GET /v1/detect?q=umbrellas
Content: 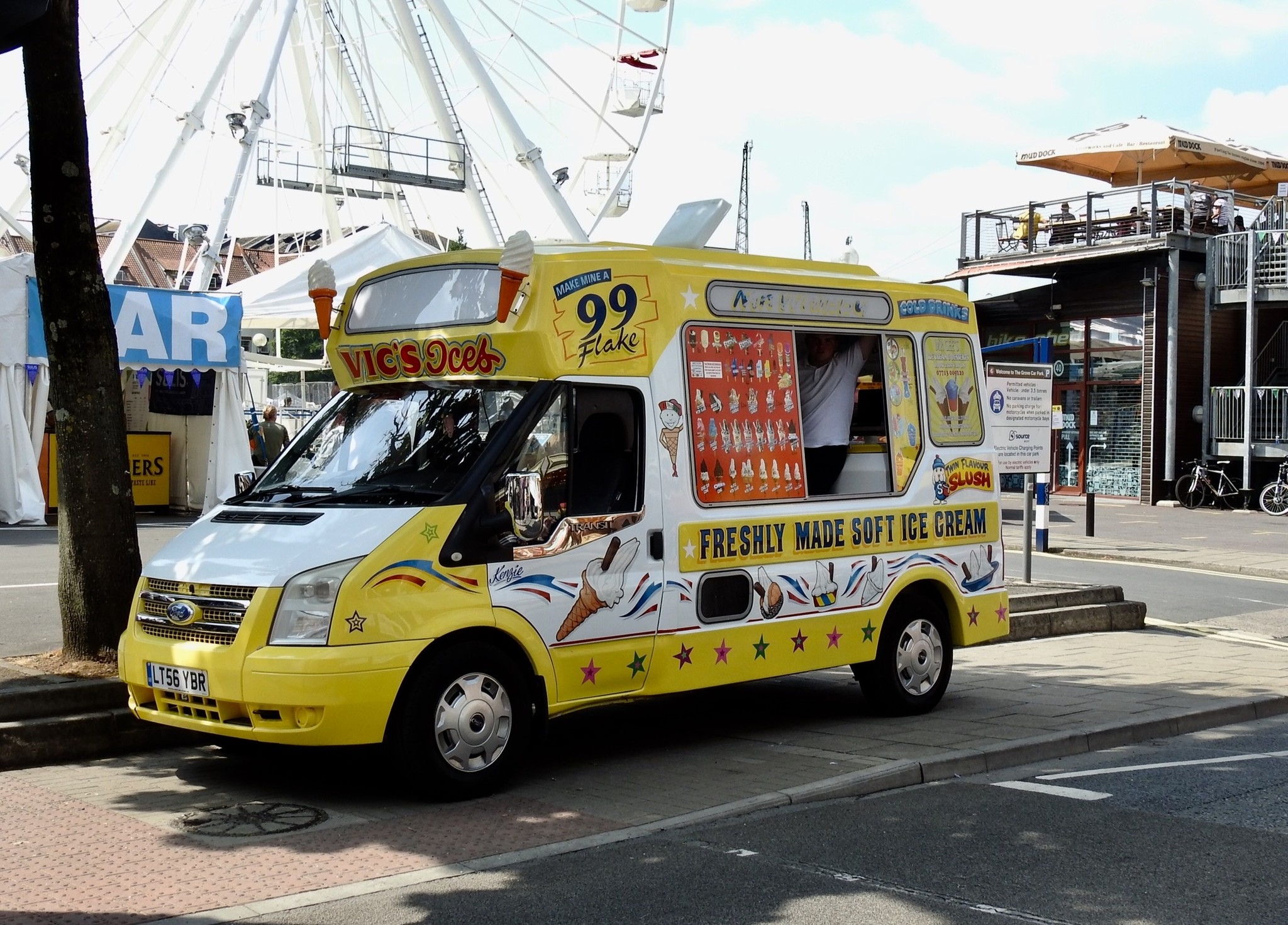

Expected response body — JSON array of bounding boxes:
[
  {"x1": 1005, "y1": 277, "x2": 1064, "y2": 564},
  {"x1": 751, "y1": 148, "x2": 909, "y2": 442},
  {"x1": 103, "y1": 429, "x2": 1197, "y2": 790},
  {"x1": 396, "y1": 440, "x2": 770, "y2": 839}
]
[{"x1": 1016, "y1": 113, "x2": 1288, "y2": 235}]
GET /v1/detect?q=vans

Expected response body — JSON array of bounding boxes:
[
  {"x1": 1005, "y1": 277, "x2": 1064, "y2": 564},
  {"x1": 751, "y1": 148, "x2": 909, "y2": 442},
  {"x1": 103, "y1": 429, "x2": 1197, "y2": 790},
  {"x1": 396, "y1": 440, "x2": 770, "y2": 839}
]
[{"x1": 118, "y1": 198, "x2": 1012, "y2": 804}]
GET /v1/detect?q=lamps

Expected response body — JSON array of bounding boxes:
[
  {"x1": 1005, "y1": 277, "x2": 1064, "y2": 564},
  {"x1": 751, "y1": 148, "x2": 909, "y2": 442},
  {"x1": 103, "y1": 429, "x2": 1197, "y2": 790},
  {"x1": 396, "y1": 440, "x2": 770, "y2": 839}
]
[
  {"x1": 1139, "y1": 278, "x2": 1155, "y2": 286},
  {"x1": 14, "y1": 153, "x2": 30, "y2": 176},
  {"x1": 552, "y1": 167, "x2": 569, "y2": 190},
  {"x1": 335, "y1": 197, "x2": 344, "y2": 210},
  {"x1": 182, "y1": 225, "x2": 209, "y2": 255},
  {"x1": 1044, "y1": 312, "x2": 1056, "y2": 321},
  {"x1": 226, "y1": 112, "x2": 248, "y2": 138},
  {"x1": 1194, "y1": 273, "x2": 1206, "y2": 290},
  {"x1": 1193, "y1": 405, "x2": 1203, "y2": 423}
]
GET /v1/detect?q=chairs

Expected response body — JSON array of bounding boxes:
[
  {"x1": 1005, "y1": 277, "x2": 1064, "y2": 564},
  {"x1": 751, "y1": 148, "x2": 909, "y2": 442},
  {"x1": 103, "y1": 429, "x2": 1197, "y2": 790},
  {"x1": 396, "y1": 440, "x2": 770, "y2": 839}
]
[
  {"x1": 996, "y1": 221, "x2": 1015, "y2": 251},
  {"x1": 484, "y1": 420, "x2": 525, "y2": 493},
  {"x1": 565, "y1": 410, "x2": 631, "y2": 515},
  {"x1": 1140, "y1": 200, "x2": 1239, "y2": 234},
  {"x1": 1049, "y1": 209, "x2": 1112, "y2": 243},
  {"x1": 1013, "y1": 220, "x2": 1037, "y2": 251}
]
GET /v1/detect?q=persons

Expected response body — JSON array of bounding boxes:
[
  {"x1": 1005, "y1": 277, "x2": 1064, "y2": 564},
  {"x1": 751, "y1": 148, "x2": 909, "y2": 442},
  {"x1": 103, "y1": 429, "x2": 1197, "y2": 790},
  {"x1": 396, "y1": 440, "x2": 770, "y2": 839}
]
[
  {"x1": 1118, "y1": 180, "x2": 1247, "y2": 237},
  {"x1": 243, "y1": 396, "x2": 292, "y2": 468},
  {"x1": 1048, "y1": 202, "x2": 1077, "y2": 246},
  {"x1": 1010, "y1": 205, "x2": 1048, "y2": 250},
  {"x1": 792, "y1": 332, "x2": 882, "y2": 498}
]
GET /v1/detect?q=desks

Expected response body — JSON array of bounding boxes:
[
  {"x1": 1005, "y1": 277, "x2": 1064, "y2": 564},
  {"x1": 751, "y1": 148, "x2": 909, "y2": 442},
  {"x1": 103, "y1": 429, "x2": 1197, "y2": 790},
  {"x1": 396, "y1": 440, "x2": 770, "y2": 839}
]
[
  {"x1": 1147, "y1": 207, "x2": 1215, "y2": 230},
  {"x1": 1038, "y1": 213, "x2": 1146, "y2": 246}
]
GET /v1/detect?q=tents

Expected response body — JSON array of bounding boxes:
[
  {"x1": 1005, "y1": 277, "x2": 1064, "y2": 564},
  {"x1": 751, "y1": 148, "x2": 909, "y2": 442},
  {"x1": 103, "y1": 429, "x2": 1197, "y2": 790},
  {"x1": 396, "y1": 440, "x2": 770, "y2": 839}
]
[{"x1": 213, "y1": 219, "x2": 441, "y2": 358}]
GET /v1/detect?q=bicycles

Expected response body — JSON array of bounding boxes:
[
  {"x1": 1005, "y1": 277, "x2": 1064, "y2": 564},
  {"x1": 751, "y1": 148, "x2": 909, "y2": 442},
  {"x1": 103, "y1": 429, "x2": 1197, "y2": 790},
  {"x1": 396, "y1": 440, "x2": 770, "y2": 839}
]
[
  {"x1": 1175, "y1": 459, "x2": 1251, "y2": 510},
  {"x1": 1259, "y1": 455, "x2": 1288, "y2": 516}
]
